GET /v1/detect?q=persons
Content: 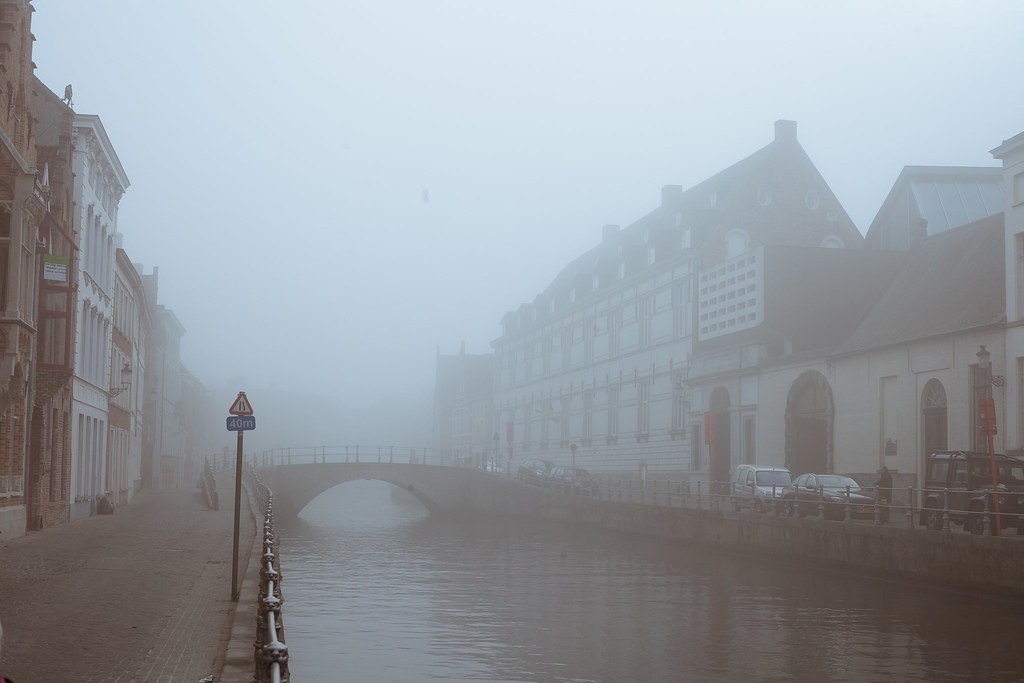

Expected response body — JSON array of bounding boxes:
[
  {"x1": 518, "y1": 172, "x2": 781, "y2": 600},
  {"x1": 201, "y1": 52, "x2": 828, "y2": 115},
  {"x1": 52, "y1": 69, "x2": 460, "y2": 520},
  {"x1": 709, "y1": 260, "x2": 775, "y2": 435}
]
[{"x1": 873, "y1": 466, "x2": 893, "y2": 522}]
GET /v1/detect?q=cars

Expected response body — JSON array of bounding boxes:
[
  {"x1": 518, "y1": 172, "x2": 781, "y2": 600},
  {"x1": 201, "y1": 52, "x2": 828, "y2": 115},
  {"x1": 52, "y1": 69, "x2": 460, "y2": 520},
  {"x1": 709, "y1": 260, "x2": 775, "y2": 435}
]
[
  {"x1": 781, "y1": 473, "x2": 876, "y2": 521},
  {"x1": 518, "y1": 458, "x2": 556, "y2": 482},
  {"x1": 550, "y1": 466, "x2": 592, "y2": 487}
]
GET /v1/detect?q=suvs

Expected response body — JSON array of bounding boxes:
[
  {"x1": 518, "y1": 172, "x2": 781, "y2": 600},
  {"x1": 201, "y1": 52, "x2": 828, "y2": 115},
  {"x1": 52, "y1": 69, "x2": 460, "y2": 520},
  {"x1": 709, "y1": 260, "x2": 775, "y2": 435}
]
[{"x1": 919, "y1": 449, "x2": 1024, "y2": 537}]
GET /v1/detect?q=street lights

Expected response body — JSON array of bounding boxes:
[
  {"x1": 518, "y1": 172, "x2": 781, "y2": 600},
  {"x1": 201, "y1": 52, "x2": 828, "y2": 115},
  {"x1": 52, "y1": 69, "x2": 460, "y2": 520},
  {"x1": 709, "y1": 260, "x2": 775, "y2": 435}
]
[{"x1": 976, "y1": 343, "x2": 1003, "y2": 537}]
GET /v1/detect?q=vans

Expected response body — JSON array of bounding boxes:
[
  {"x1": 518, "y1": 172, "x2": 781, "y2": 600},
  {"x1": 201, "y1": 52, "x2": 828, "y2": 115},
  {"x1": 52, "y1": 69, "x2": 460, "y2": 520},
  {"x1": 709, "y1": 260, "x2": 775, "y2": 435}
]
[{"x1": 731, "y1": 464, "x2": 794, "y2": 511}]
